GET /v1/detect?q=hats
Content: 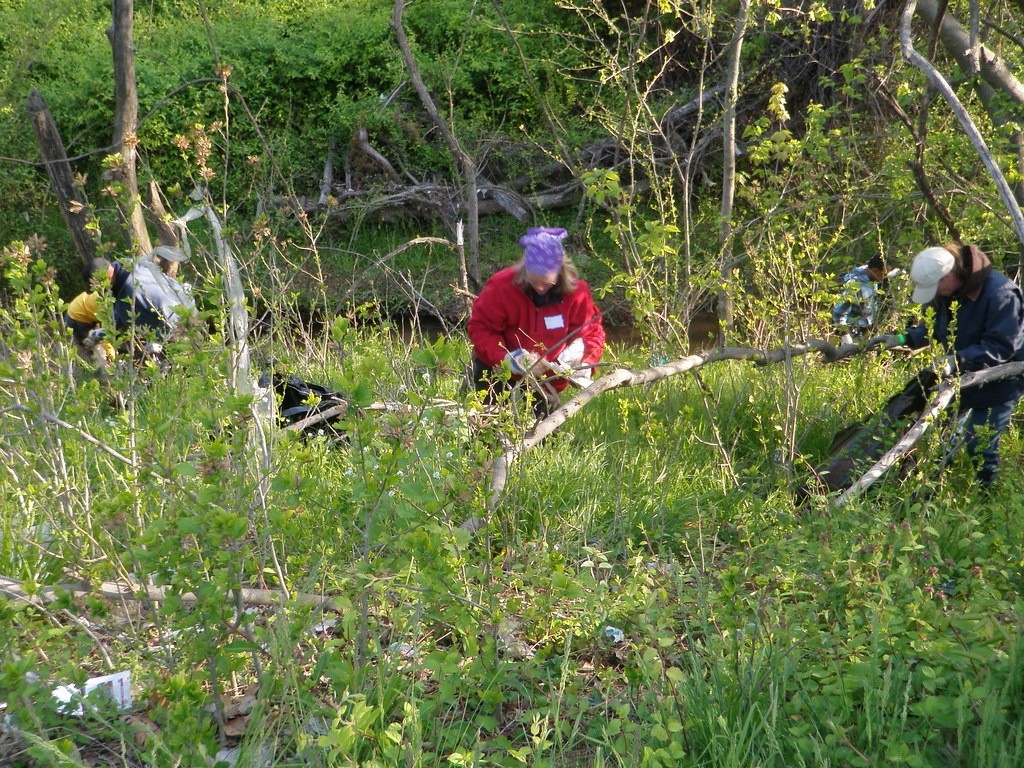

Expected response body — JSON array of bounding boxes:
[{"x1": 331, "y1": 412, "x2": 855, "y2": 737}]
[
  {"x1": 909, "y1": 248, "x2": 955, "y2": 303},
  {"x1": 84, "y1": 258, "x2": 111, "y2": 294},
  {"x1": 521, "y1": 226, "x2": 569, "y2": 272}
]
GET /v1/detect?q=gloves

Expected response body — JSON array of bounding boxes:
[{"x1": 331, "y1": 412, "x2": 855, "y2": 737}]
[
  {"x1": 867, "y1": 333, "x2": 906, "y2": 353},
  {"x1": 569, "y1": 363, "x2": 593, "y2": 390},
  {"x1": 504, "y1": 348, "x2": 547, "y2": 378},
  {"x1": 931, "y1": 355, "x2": 958, "y2": 376}
]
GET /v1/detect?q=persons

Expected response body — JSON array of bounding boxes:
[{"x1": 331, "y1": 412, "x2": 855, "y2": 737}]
[
  {"x1": 454, "y1": 226, "x2": 605, "y2": 421},
  {"x1": 64, "y1": 254, "x2": 199, "y2": 345},
  {"x1": 866, "y1": 244, "x2": 1024, "y2": 503},
  {"x1": 831, "y1": 253, "x2": 893, "y2": 339}
]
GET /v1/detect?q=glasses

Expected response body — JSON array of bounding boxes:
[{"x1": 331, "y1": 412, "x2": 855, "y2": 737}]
[{"x1": 529, "y1": 278, "x2": 557, "y2": 287}]
